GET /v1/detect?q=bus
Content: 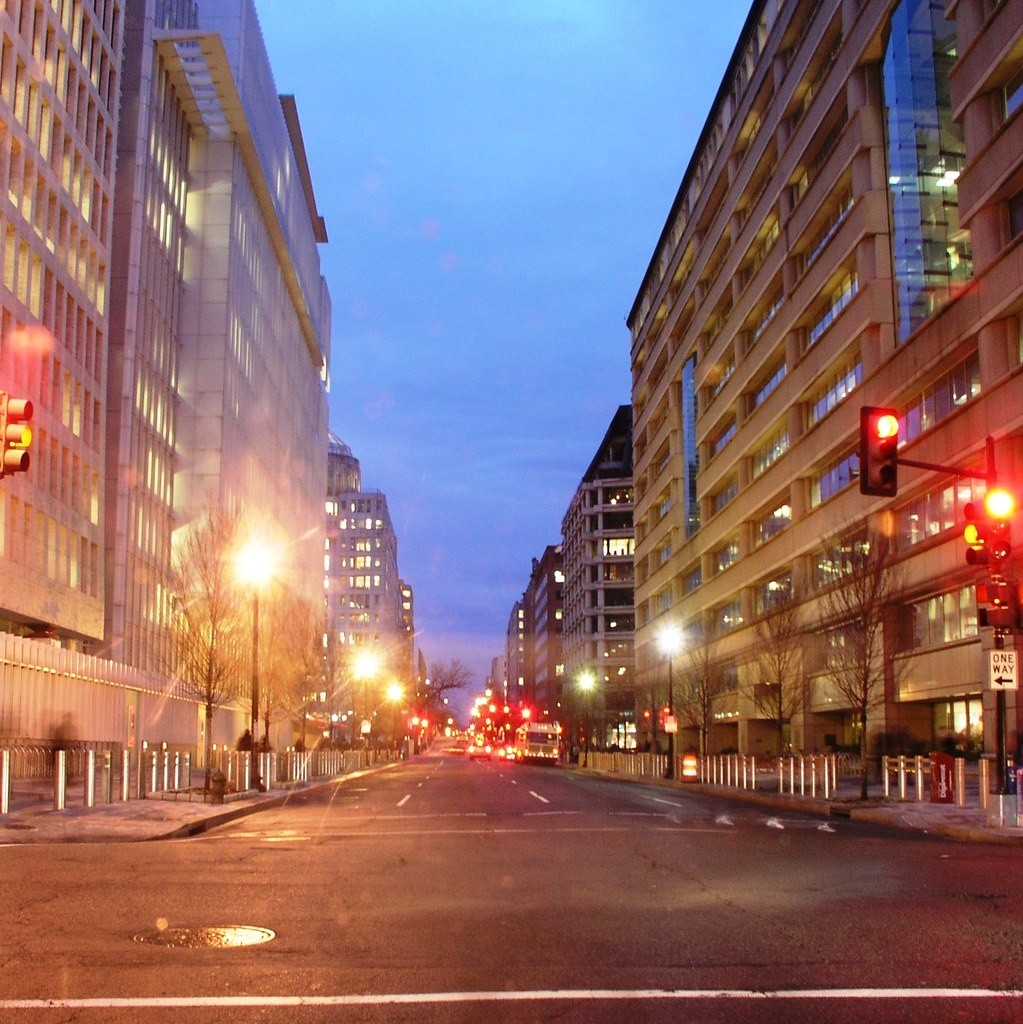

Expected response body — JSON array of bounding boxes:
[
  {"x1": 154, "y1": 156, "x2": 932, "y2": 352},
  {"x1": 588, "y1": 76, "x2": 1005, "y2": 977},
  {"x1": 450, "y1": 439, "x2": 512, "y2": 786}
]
[{"x1": 514, "y1": 722, "x2": 559, "y2": 767}]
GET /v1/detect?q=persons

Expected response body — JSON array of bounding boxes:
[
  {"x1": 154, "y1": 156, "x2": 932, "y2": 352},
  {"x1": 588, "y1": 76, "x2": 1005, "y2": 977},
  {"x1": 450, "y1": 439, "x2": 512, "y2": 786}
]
[
  {"x1": 237, "y1": 727, "x2": 426, "y2": 757},
  {"x1": 870, "y1": 725, "x2": 1023, "y2": 794},
  {"x1": 570, "y1": 738, "x2": 739, "y2": 763}
]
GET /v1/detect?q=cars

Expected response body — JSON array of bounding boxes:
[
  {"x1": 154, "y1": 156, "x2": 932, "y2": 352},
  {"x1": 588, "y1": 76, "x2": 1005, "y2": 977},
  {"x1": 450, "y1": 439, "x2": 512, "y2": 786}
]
[
  {"x1": 470, "y1": 739, "x2": 492, "y2": 761},
  {"x1": 498, "y1": 744, "x2": 516, "y2": 760}
]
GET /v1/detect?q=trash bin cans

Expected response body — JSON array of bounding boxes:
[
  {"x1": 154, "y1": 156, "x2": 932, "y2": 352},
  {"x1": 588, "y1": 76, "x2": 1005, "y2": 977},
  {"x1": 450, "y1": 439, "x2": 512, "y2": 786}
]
[{"x1": 929, "y1": 750, "x2": 955, "y2": 804}]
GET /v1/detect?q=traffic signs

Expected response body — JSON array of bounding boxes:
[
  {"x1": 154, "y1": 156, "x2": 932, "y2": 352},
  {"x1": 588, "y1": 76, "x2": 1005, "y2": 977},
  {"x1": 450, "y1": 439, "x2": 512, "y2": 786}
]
[{"x1": 988, "y1": 648, "x2": 1020, "y2": 690}]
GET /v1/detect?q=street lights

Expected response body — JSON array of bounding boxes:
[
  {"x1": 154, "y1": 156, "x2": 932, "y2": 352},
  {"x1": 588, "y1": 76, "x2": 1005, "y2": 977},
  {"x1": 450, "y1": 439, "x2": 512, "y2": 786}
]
[
  {"x1": 240, "y1": 542, "x2": 275, "y2": 790},
  {"x1": 657, "y1": 627, "x2": 681, "y2": 776},
  {"x1": 580, "y1": 672, "x2": 595, "y2": 764},
  {"x1": 357, "y1": 657, "x2": 377, "y2": 766},
  {"x1": 389, "y1": 684, "x2": 401, "y2": 742}
]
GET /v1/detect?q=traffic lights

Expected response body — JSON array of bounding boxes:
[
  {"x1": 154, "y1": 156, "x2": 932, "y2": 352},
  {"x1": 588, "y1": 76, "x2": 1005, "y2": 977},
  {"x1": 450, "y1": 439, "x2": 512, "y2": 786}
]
[
  {"x1": 984, "y1": 492, "x2": 1016, "y2": 561},
  {"x1": 0, "y1": 390, "x2": 33, "y2": 480},
  {"x1": 963, "y1": 500, "x2": 990, "y2": 569},
  {"x1": 861, "y1": 405, "x2": 901, "y2": 499},
  {"x1": 974, "y1": 579, "x2": 1022, "y2": 629}
]
